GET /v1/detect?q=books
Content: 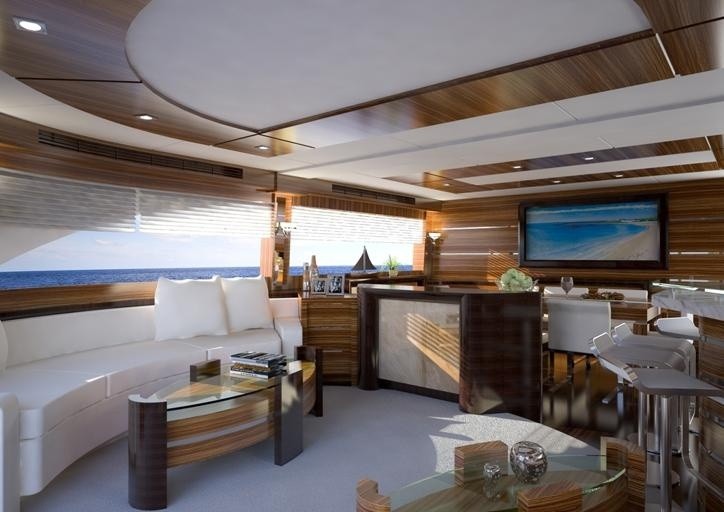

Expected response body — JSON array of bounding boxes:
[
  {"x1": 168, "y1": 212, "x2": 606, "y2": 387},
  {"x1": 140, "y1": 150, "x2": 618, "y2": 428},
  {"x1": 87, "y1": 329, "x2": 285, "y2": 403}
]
[{"x1": 228, "y1": 350, "x2": 287, "y2": 380}]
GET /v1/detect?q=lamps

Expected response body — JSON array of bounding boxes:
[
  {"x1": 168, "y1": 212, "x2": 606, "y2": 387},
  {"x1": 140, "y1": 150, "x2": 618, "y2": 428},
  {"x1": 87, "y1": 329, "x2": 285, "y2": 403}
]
[{"x1": 429, "y1": 232, "x2": 441, "y2": 275}]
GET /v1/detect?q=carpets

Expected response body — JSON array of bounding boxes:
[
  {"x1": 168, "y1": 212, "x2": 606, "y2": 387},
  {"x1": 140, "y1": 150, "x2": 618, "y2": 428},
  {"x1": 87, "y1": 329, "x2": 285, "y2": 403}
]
[{"x1": 19, "y1": 385, "x2": 682, "y2": 512}]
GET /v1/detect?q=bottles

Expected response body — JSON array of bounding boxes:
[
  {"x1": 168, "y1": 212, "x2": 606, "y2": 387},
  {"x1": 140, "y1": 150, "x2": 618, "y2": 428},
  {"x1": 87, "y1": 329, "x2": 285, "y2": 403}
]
[
  {"x1": 303, "y1": 255, "x2": 320, "y2": 291},
  {"x1": 275, "y1": 252, "x2": 285, "y2": 288}
]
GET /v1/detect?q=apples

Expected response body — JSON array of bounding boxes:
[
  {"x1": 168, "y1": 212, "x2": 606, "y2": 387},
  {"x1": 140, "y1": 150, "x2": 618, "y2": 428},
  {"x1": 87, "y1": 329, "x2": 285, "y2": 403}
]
[{"x1": 501, "y1": 268, "x2": 533, "y2": 292}]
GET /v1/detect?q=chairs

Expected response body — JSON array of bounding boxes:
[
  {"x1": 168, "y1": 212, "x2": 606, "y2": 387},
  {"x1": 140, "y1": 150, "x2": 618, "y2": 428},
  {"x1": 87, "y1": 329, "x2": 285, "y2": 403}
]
[{"x1": 547, "y1": 298, "x2": 611, "y2": 381}]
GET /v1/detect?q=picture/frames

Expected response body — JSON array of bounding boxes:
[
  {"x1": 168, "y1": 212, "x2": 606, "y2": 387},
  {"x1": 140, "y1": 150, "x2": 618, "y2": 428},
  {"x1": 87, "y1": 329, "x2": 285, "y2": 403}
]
[
  {"x1": 311, "y1": 278, "x2": 327, "y2": 294},
  {"x1": 517, "y1": 192, "x2": 670, "y2": 271},
  {"x1": 327, "y1": 273, "x2": 344, "y2": 295}
]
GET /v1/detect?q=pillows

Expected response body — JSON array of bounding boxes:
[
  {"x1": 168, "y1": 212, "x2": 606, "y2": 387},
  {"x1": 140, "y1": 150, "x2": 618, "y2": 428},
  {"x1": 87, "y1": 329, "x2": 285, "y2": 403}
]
[
  {"x1": 221, "y1": 275, "x2": 274, "y2": 333},
  {"x1": 154, "y1": 276, "x2": 228, "y2": 340}
]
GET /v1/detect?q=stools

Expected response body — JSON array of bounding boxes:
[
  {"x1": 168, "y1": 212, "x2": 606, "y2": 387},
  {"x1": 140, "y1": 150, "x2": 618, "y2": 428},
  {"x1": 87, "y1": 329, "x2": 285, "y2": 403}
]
[{"x1": 589, "y1": 316, "x2": 723, "y2": 512}]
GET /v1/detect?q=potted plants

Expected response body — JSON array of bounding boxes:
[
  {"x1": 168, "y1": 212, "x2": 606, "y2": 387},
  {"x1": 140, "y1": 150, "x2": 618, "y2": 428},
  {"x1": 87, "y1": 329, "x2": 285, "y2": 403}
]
[{"x1": 387, "y1": 256, "x2": 398, "y2": 277}]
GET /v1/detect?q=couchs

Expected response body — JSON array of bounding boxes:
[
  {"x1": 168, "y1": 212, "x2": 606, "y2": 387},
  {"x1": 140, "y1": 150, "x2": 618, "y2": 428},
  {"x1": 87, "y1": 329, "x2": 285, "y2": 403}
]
[{"x1": 0, "y1": 298, "x2": 303, "y2": 512}]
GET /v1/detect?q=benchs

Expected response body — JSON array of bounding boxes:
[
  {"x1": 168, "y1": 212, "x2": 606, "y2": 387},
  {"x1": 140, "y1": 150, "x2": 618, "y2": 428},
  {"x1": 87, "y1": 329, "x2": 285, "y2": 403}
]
[{"x1": 533, "y1": 285, "x2": 648, "y2": 302}]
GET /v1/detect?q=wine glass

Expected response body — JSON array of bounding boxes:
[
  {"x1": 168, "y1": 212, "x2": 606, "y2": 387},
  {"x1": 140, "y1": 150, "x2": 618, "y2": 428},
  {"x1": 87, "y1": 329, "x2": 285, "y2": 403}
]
[{"x1": 560, "y1": 277, "x2": 573, "y2": 298}]
